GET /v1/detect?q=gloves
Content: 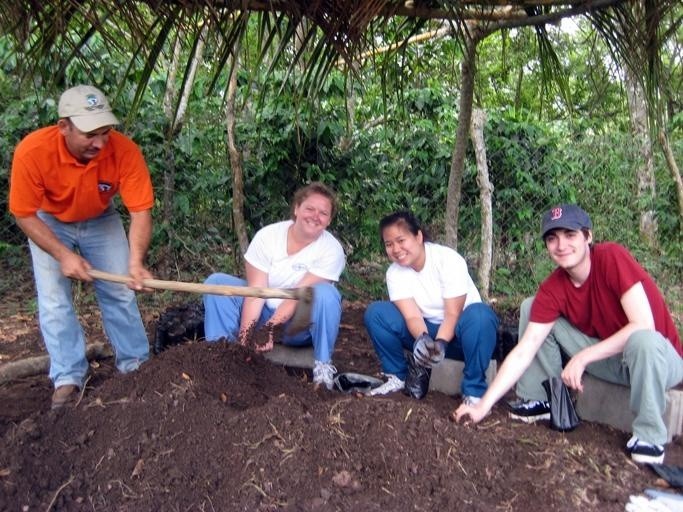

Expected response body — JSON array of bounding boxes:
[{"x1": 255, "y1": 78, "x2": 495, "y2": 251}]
[{"x1": 411, "y1": 332, "x2": 448, "y2": 370}]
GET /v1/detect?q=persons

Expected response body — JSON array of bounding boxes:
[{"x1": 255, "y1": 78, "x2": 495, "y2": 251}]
[
  {"x1": 6, "y1": 83, "x2": 164, "y2": 408},
  {"x1": 201, "y1": 181, "x2": 346, "y2": 391},
  {"x1": 361, "y1": 210, "x2": 500, "y2": 417},
  {"x1": 451, "y1": 203, "x2": 683, "y2": 466}
]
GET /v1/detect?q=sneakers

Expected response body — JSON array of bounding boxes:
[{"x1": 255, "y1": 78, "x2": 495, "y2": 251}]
[
  {"x1": 50, "y1": 385, "x2": 81, "y2": 409},
  {"x1": 626, "y1": 434, "x2": 665, "y2": 464},
  {"x1": 312, "y1": 361, "x2": 337, "y2": 401},
  {"x1": 452, "y1": 395, "x2": 492, "y2": 420},
  {"x1": 364, "y1": 371, "x2": 407, "y2": 404},
  {"x1": 507, "y1": 398, "x2": 551, "y2": 424}
]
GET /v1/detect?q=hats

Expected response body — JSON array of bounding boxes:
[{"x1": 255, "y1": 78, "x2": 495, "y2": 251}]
[
  {"x1": 540, "y1": 206, "x2": 590, "y2": 240},
  {"x1": 57, "y1": 84, "x2": 120, "y2": 134}
]
